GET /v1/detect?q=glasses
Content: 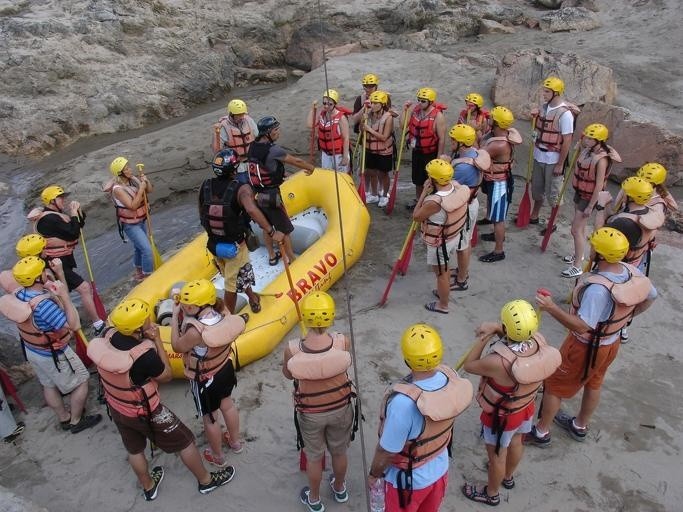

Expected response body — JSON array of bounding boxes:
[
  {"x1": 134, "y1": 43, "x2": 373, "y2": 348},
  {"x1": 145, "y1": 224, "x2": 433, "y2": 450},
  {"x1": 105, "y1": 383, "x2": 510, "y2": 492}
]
[
  {"x1": 323, "y1": 102, "x2": 333, "y2": 105},
  {"x1": 417, "y1": 98, "x2": 428, "y2": 103}
]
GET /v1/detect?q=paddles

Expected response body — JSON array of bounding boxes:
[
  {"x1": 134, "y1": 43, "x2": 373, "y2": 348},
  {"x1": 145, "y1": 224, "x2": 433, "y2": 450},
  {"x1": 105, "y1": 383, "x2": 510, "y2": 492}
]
[
  {"x1": 76, "y1": 211, "x2": 107, "y2": 322},
  {"x1": 516, "y1": 112, "x2": 537, "y2": 227},
  {"x1": 54, "y1": 294, "x2": 94, "y2": 371},
  {"x1": 387, "y1": 107, "x2": 408, "y2": 214},
  {"x1": 140, "y1": 170, "x2": 162, "y2": 272},
  {"x1": 358, "y1": 108, "x2": 368, "y2": 205},
  {"x1": 542, "y1": 136, "x2": 581, "y2": 251},
  {"x1": 400, "y1": 184, "x2": 430, "y2": 274},
  {"x1": 1, "y1": 371, "x2": 26, "y2": 413}
]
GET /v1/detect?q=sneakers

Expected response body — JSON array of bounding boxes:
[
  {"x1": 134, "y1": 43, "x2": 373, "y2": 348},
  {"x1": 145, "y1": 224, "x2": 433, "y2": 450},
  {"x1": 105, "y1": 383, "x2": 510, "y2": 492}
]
[
  {"x1": 198, "y1": 465, "x2": 235, "y2": 495},
  {"x1": 406, "y1": 198, "x2": 418, "y2": 210},
  {"x1": 4, "y1": 421, "x2": 25, "y2": 444},
  {"x1": 366, "y1": 194, "x2": 379, "y2": 204},
  {"x1": 480, "y1": 232, "x2": 504, "y2": 242},
  {"x1": 562, "y1": 253, "x2": 585, "y2": 264},
  {"x1": 540, "y1": 223, "x2": 558, "y2": 236},
  {"x1": 203, "y1": 447, "x2": 228, "y2": 468},
  {"x1": 300, "y1": 485, "x2": 326, "y2": 512},
  {"x1": 89, "y1": 324, "x2": 114, "y2": 338},
  {"x1": 69, "y1": 414, "x2": 103, "y2": 434},
  {"x1": 620, "y1": 326, "x2": 632, "y2": 344},
  {"x1": 480, "y1": 252, "x2": 506, "y2": 263},
  {"x1": 142, "y1": 464, "x2": 164, "y2": 502},
  {"x1": 560, "y1": 266, "x2": 583, "y2": 278},
  {"x1": 477, "y1": 217, "x2": 492, "y2": 225},
  {"x1": 378, "y1": 191, "x2": 391, "y2": 199},
  {"x1": 377, "y1": 196, "x2": 389, "y2": 208},
  {"x1": 248, "y1": 293, "x2": 262, "y2": 313},
  {"x1": 329, "y1": 472, "x2": 350, "y2": 503},
  {"x1": 60, "y1": 406, "x2": 86, "y2": 430},
  {"x1": 223, "y1": 430, "x2": 244, "y2": 454},
  {"x1": 364, "y1": 191, "x2": 376, "y2": 197},
  {"x1": 514, "y1": 216, "x2": 540, "y2": 225},
  {"x1": 134, "y1": 272, "x2": 144, "y2": 281},
  {"x1": 237, "y1": 312, "x2": 250, "y2": 324},
  {"x1": 553, "y1": 413, "x2": 588, "y2": 443},
  {"x1": 521, "y1": 426, "x2": 551, "y2": 449}
]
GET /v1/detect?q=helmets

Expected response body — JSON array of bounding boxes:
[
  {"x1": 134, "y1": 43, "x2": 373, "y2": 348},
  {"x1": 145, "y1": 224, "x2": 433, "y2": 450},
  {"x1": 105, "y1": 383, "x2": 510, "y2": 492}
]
[
  {"x1": 226, "y1": 98, "x2": 248, "y2": 115},
  {"x1": 324, "y1": 90, "x2": 340, "y2": 105},
  {"x1": 209, "y1": 148, "x2": 239, "y2": 176},
  {"x1": 255, "y1": 114, "x2": 281, "y2": 134},
  {"x1": 110, "y1": 155, "x2": 129, "y2": 178},
  {"x1": 402, "y1": 323, "x2": 444, "y2": 372},
  {"x1": 543, "y1": 76, "x2": 565, "y2": 96},
  {"x1": 369, "y1": 90, "x2": 389, "y2": 105},
  {"x1": 13, "y1": 256, "x2": 46, "y2": 288},
  {"x1": 621, "y1": 176, "x2": 654, "y2": 205},
  {"x1": 465, "y1": 92, "x2": 485, "y2": 108},
  {"x1": 416, "y1": 87, "x2": 437, "y2": 102},
  {"x1": 425, "y1": 158, "x2": 455, "y2": 186},
  {"x1": 111, "y1": 295, "x2": 150, "y2": 336},
  {"x1": 360, "y1": 72, "x2": 381, "y2": 87},
  {"x1": 449, "y1": 124, "x2": 476, "y2": 147},
  {"x1": 179, "y1": 279, "x2": 218, "y2": 307},
  {"x1": 583, "y1": 122, "x2": 609, "y2": 143},
  {"x1": 590, "y1": 228, "x2": 630, "y2": 264},
  {"x1": 41, "y1": 183, "x2": 71, "y2": 204},
  {"x1": 300, "y1": 290, "x2": 338, "y2": 329},
  {"x1": 501, "y1": 299, "x2": 539, "y2": 342},
  {"x1": 637, "y1": 162, "x2": 667, "y2": 185},
  {"x1": 491, "y1": 106, "x2": 513, "y2": 129},
  {"x1": 16, "y1": 232, "x2": 46, "y2": 258}
]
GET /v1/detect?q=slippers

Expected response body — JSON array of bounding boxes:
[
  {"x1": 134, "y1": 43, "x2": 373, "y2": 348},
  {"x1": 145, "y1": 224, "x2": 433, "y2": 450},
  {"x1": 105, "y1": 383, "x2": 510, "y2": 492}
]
[
  {"x1": 425, "y1": 302, "x2": 449, "y2": 314},
  {"x1": 432, "y1": 288, "x2": 440, "y2": 299}
]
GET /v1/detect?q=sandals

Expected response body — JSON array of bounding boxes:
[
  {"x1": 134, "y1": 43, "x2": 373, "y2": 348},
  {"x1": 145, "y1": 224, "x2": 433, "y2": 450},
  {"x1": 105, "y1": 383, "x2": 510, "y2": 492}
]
[
  {"x1": 448, "y1": 277, "x2": 468, "y2": 291},
  {"x1": 462, "y1": 483, "x2": 502, "y2": 507},
  {"x1": 269, "y1": 251, "x2": 282, "y2": 266},
  {"x1": 448, "y1": 266, "x2": 470, "y2": 279},
  {"x1": 486, "y1": 460, "x2": 515, "y2": 490}
]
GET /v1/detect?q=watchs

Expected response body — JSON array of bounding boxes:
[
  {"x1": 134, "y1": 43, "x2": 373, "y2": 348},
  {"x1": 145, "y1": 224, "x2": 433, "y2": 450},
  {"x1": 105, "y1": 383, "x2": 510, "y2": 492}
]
[
  {"x1": 268, "y1": 225, "x2": 276, "y2": 238},
  {"x1": 596, "y1": 203, "x2": 605, "y2": 211}
]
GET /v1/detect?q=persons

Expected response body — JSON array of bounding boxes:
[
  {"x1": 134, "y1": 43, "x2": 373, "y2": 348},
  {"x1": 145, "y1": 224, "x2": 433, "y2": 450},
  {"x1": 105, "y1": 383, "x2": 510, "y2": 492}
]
[
  {"x1": 102, "y1": 156, "x2": 154, "y2": 282},
  {"x1": 213, "y1": 99, "x2": 259, "y2": 184},
  {"x1": 359, "y1": 90, "x2": 400, "y2": 207},
  {"x1": 351, "y1": 73, "x2": 392, "y2": 199},
  {"x1": 412, "y1": 158, "x2": 470, "y2": 314},
  {"x1": 457, "y1": 93, "x2": 483, "y2": 127},
  {"x1": 170, "y1": 278, "x2": 245, "y2": 468},
  {"x1": 474, "y1": 106, "x2": 495, "y2": 225},
  {"x1": 462, "y1": 299, "x2": 562, "y2": 506},
  {"x1": 198, "y1": 147, "x2": 285, "y2": 323},
  {"x1": 521, "y1": 227, "x2": 658, "y2": 450},
  {"x1": 438, "y1": 126, "x2": 490, "y2": 291},
  {"x1": 594, "y1": 178, "x2": 665, "y2": 344},
  {"x1": 87, "y1": 298, "x2": 236, "y2": 502},
  {"x1": 615, "y1": 163, "x2": 677, "y2": 213},
  {"x1": 560, "y1": 123, "x2": 622, "y2": 278},
  {"x1": 512, "y1": 76, "x2": 582, "y2": 233},
  {"x1": 306, "y1": 88, "x2": 353, "y2": 175},
  {"x1": 27, "y1": 185, "x2": 106, "y2": 336},
  {"x1": 0, "y1": 232, "x2": 69, "y2": 297},
  {"x1": 283, "y1": 290, "x2": 359, "y2": 512},
  {"x1": 399, "y1": 87, "x2": 447, "y2": 209},
  {"x1": 0, "y1": 256, "x2": 103, "y2": 434},
  {"x1": 246, "y1": 115, "x2": 314, "y2": 266},
  {"x1": 478, "y1": 105, "x2": 522, "y2": 262},
  {"x1": 0, "y1": 382, "x2": 26, "y2": 444},
  {"x1": 367, "y1": 321, "x2": 473, "y2": 512}
]
